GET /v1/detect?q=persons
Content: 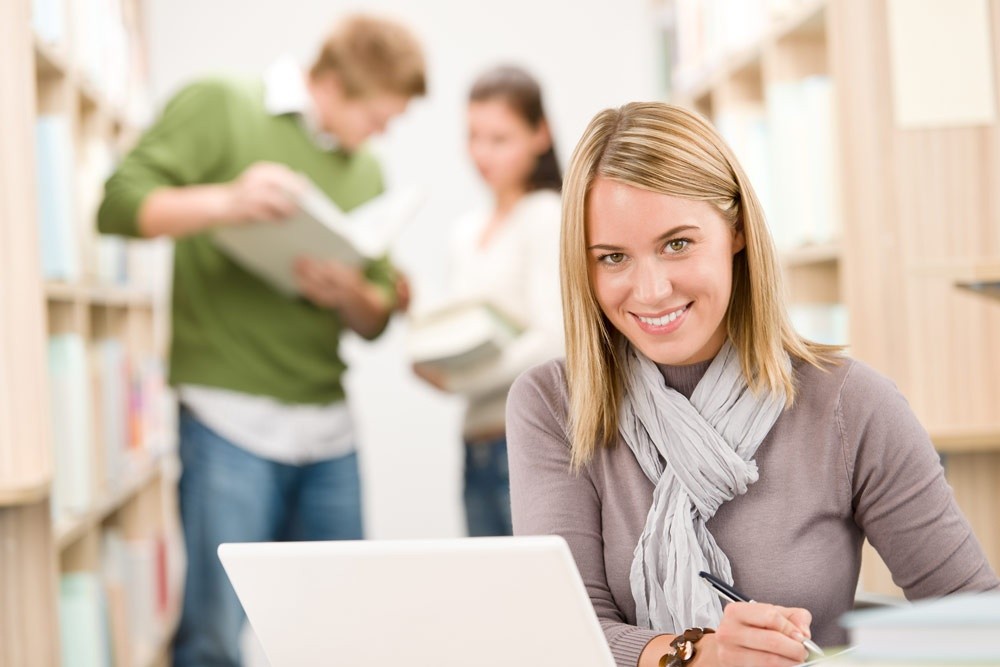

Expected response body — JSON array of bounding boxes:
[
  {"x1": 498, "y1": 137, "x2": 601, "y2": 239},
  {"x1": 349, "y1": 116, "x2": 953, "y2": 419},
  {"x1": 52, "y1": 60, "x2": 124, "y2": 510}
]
[
  {"x1": 394, "y1": 64, "x2": 570, "y2": 538},
  {"x1": 504, "y1": 102, "x2": 1000, "y2": 667},
  {"x1": 96, "y1": 17, "x2": 430, "y2": 667}
]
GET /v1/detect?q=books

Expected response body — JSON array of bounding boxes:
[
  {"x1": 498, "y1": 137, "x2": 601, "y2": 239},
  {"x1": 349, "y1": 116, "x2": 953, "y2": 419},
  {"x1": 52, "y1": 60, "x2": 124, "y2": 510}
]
[
  {"x1": 216, "y1": 159, "x2": 387, "y2": 303},
  {"x1": 405, "y1": 284, "x2": 529, "y2": 388},
  {"x1": 840, "y1": 593, "x2": 1000, "y2": 663}
]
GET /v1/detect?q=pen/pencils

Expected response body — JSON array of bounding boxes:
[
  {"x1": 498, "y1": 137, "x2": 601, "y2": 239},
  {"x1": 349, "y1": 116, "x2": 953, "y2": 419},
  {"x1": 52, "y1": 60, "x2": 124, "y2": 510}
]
[{"x1": 699, "y1": 572, "x2": 825, "y2": 656}]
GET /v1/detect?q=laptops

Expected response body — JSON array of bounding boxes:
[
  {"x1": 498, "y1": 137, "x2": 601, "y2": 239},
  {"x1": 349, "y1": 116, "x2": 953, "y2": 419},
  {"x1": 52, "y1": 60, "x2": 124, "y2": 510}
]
[{"x1": 216, "y1": 533, "x2": 618, "y2": 667}]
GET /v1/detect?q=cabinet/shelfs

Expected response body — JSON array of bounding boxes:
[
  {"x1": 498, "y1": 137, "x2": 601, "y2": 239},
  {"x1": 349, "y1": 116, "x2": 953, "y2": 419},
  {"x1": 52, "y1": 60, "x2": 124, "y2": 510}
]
[
  {"x1": 654, "y1": 0, "x2": 1000, "y2": 606},
  {"x1": 0, "y1": 1, "x2": 197, "y2": 667}
]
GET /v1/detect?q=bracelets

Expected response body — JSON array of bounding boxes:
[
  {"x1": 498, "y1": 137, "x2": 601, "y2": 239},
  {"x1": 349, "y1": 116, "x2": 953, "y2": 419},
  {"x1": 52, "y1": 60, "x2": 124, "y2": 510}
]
[{"x1": 657, "y1": 626, "x2": 715, "y2": 667}]
